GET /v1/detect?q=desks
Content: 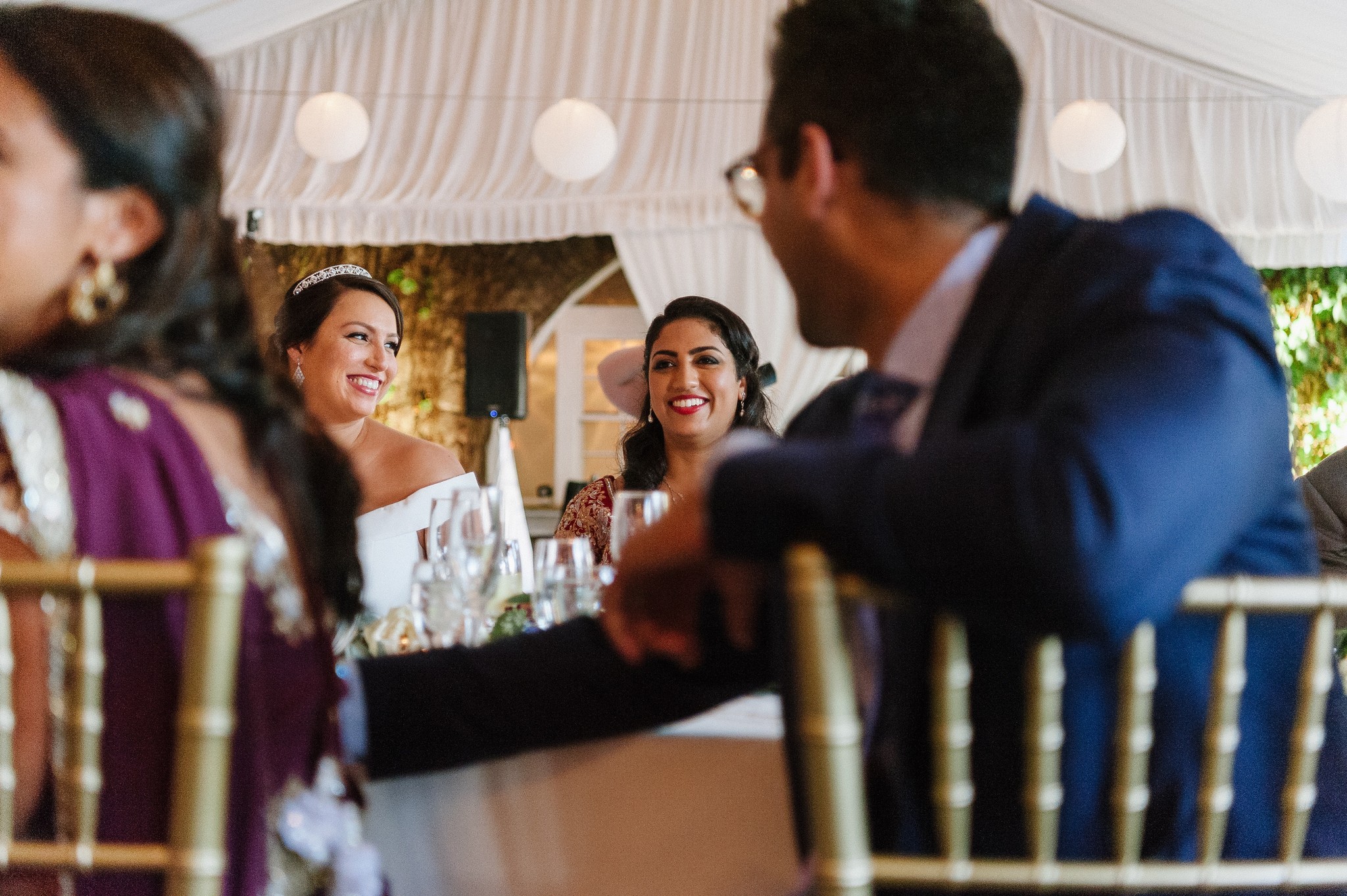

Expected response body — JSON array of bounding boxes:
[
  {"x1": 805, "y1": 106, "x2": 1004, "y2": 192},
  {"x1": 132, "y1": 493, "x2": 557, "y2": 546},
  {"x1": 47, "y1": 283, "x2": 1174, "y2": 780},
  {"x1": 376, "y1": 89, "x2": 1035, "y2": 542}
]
[{"x1": 362, "y1": 693, "x2": 802, "y2": 896}]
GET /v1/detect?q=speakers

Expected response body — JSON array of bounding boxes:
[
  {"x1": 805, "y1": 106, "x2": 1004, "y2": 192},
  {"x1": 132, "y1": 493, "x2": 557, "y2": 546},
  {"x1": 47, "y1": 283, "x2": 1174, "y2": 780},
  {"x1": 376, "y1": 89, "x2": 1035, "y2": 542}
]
[{"x1": 463, "y1": 310, "x2": 528, "y2": 420}]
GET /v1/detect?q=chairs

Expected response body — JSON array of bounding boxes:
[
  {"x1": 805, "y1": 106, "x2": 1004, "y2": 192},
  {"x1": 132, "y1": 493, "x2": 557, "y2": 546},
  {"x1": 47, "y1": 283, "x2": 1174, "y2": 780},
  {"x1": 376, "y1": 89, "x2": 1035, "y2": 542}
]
[
  {"x1": 789, "y1": 543, "x2": 1347, "y2": 896},
  {"x1": 0, "y1": 533, "x2": 245, "y2": 896}
]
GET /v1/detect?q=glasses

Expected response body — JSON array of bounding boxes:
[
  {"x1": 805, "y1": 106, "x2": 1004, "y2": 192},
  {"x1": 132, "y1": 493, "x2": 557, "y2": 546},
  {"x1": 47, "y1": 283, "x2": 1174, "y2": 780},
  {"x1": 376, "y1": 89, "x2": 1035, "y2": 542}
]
[{"x1": 723, "y1": 131, "x2": 844, "y2": 222}]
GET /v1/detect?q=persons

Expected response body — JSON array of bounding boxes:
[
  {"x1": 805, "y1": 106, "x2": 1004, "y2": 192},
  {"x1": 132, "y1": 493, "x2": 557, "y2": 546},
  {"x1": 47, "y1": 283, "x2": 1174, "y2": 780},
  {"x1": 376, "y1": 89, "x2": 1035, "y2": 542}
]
[
  {"x1": 551, "y1": 296, "x2": 783, "y2": 620},
  {"x1": 1, "y1": 0, "x2": 394, "y2": 896},
  {"x1": 267, "y1": 264, "x2": 503, "y2": 643},
  {"x1": 350, "y1": 0, "x2": 1347, "y2": 896},
  {"x1": 1290, "y1": 450, "x2": 1347, "y2": 631}
]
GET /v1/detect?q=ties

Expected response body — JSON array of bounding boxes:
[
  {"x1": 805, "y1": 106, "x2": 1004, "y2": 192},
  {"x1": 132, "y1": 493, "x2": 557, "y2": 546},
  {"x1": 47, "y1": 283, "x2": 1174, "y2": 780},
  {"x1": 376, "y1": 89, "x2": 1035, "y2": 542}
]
[{"x1": 836, "y1": 375, "x2": 914, "y2": 737}]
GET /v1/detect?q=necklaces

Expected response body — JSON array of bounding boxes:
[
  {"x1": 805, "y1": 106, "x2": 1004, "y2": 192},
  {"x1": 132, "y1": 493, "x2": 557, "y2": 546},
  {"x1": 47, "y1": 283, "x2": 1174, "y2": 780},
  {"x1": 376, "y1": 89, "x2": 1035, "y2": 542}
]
[{"x1": 660, "y1": 475, "x2": 684, "y2": 506}]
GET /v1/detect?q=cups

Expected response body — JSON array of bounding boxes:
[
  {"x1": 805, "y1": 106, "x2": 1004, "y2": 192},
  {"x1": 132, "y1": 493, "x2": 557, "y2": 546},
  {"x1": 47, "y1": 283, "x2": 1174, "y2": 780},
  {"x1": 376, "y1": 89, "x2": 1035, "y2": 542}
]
[
  {"x1": 425, "y1": 496, "x2": 450, "y2": 578},
  {"x1": 610, "y1": 490, "x2": 671, "y2": 561},
  {"x1": 533, "y1": 540, "x2": 602, "y2": 631},
  {"x1": 409, "y1": 557, "x2": 475, "y2": 649}
]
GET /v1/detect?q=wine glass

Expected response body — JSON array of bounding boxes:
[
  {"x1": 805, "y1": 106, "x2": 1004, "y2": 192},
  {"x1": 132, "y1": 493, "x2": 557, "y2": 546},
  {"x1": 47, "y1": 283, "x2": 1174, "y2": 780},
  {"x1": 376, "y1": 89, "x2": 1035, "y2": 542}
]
[{"x1": 450, "y1": 484, "x2": 504, "y2": 648}]
